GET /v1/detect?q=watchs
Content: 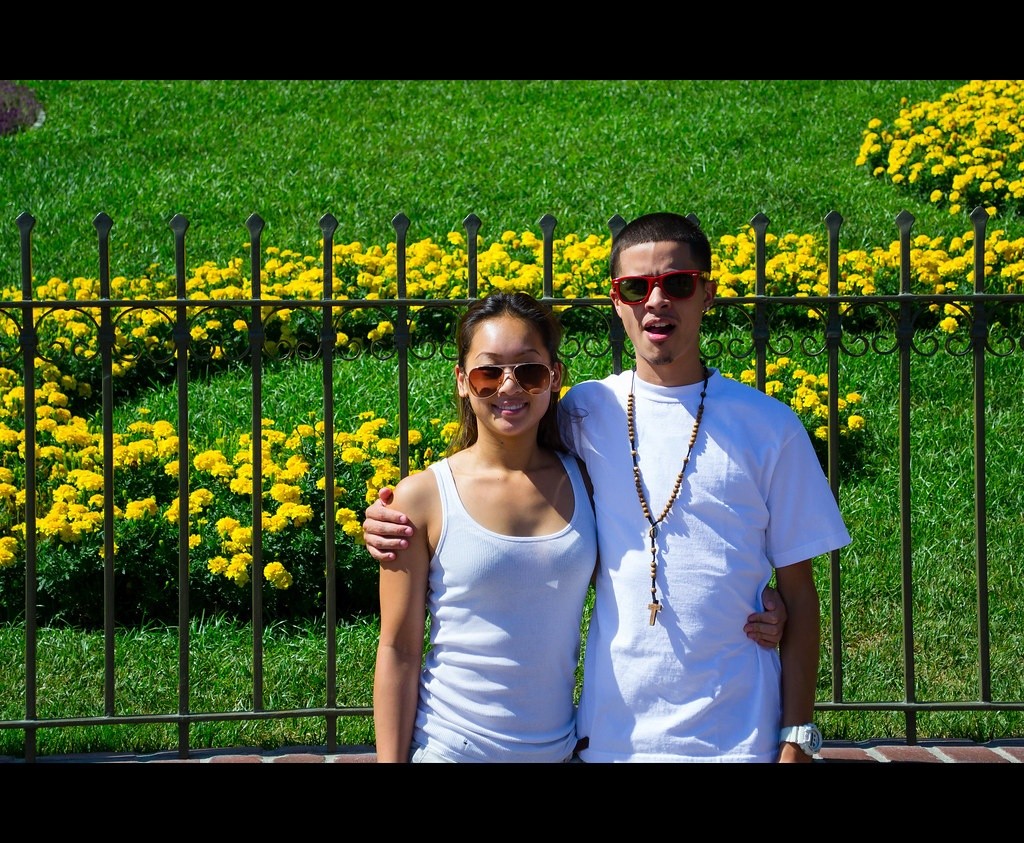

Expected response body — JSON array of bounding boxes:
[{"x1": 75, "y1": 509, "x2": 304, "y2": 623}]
[{"x1": 778, "y1": 723, "x2": 824, "y2": 757}]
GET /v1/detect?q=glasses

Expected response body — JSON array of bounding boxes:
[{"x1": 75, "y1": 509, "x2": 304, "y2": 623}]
[
  {"x1": 611, "y1": 269, "x2": 699, "y2": 305},
  {"x1": 461, "y1": 363, "x2": 555, "y2": 398}
]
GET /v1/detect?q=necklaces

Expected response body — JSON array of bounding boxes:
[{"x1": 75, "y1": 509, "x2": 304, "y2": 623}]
[{"x1": 625, "y1": 356, "x2": 710, "y2": 626}]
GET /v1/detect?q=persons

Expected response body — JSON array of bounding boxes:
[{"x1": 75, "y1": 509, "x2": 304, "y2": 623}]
[
  {"x1": 370, "y1": 288, "x2": 791, "y2": 765},
  {"x1": 358, "y1": 211, "x2": 852, "y2": 765}
]
